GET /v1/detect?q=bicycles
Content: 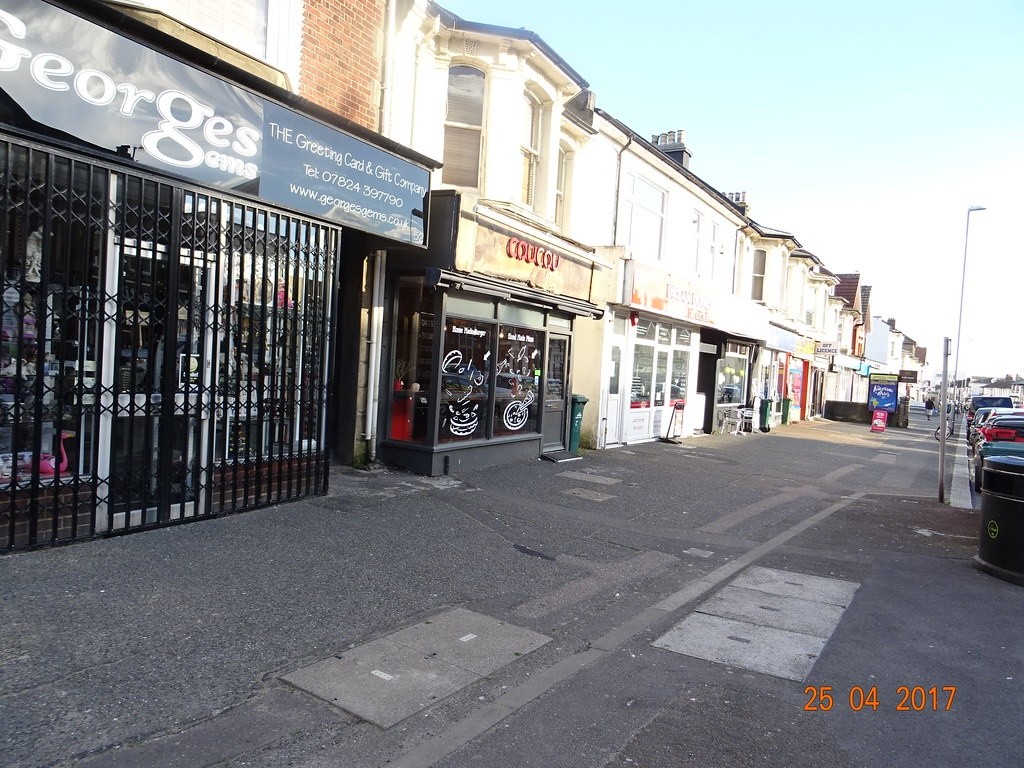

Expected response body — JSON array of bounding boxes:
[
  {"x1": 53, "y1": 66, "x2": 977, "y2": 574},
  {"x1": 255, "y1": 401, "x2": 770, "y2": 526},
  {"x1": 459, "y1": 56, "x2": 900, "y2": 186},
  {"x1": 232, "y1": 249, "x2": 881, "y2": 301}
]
[{"x1": 935, "y1": 416, "x2": 954, "y2": 440}]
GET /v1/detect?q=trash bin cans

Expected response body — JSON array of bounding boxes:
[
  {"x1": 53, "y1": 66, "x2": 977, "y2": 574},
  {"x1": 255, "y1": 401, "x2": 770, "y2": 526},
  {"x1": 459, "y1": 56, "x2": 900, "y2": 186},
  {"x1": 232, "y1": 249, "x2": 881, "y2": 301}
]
[
  {"x1": 760, "y1": 400, "x2": 773, "y2": 433},
  {"x1": 970, "y1": 441, "x2": 1024, "y2": 587},
  {"x1": 782, "y1": 398, "x2": 791, "y2": 425},
  {"x1": 569, "y1": 394, "x2": 589, "y2": 453}
]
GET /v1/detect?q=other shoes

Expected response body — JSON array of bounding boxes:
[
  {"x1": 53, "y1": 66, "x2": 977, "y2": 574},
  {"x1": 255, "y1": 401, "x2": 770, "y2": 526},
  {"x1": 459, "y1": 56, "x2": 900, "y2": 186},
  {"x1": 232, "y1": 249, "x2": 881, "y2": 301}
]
[{"x1": 928, "y1": 419, "x2": 930, "y2": 421}]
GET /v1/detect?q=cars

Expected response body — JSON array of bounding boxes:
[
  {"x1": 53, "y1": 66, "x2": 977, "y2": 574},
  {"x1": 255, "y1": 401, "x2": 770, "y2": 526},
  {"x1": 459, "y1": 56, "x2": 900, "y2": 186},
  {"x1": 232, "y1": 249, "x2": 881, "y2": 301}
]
[
  {"x1": 3, "y1": 334, "x2": 307, "y2": 403},
  {"x1": 967, "y1": 392, "x2": 1024, "y2": 496}
]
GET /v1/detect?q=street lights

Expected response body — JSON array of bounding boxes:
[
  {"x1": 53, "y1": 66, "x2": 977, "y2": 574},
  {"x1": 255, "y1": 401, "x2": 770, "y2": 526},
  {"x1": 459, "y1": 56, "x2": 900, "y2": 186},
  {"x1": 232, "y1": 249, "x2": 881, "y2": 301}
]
[{"x1": 951, "y1": 206, "x2": 990, "y2": 434}]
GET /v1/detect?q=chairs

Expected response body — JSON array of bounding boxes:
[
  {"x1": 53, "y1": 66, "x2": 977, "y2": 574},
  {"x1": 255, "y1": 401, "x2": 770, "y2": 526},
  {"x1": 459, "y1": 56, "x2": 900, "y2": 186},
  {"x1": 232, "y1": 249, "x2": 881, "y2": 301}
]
[{"x1": 719, "y1": 408, "x2": 757, "y2": 436}]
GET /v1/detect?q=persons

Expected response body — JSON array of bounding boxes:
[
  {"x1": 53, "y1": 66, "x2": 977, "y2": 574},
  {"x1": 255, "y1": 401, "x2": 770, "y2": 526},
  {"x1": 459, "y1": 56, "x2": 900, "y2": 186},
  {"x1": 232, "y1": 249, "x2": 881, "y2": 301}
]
[
  {"x1": 27, "y1": 224, "x2": 54, "y2": 277},
  {"x1": 925, "y1": 397, "x2": 936, "y2": 421},
  {"x1": 947, "y1": 400, "x2": 951, "y2": 413}
]
